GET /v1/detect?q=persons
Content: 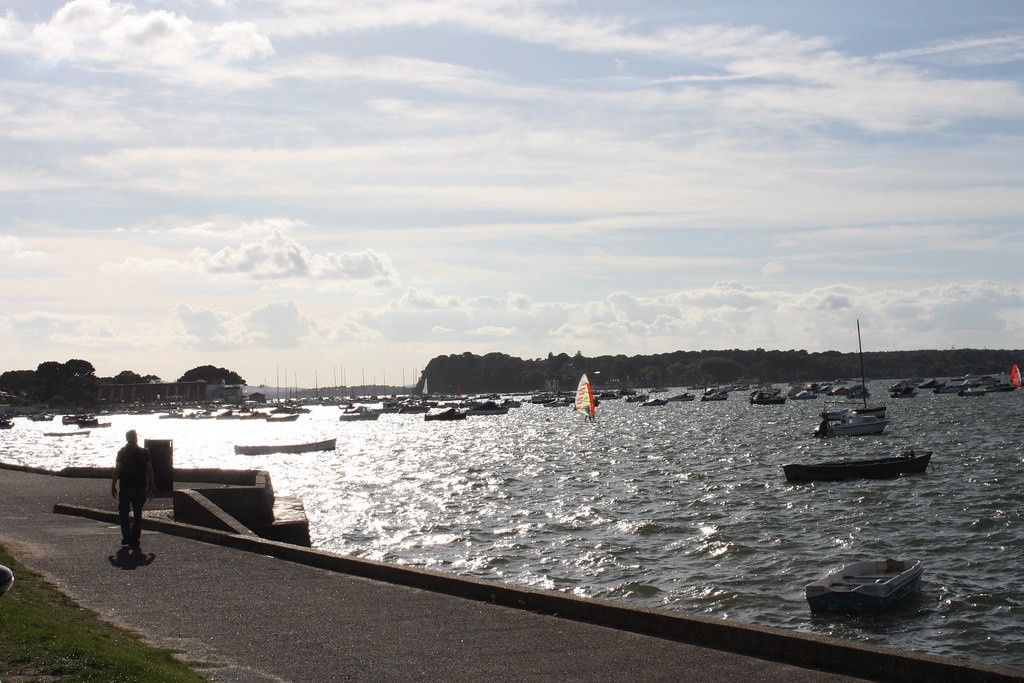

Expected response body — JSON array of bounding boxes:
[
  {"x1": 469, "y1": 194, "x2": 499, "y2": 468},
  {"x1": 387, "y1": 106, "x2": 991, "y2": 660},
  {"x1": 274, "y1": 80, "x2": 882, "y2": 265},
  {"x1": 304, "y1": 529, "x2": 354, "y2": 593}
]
[{"x1": 111, "y1": 430, "x2": 156, "y2": 546}]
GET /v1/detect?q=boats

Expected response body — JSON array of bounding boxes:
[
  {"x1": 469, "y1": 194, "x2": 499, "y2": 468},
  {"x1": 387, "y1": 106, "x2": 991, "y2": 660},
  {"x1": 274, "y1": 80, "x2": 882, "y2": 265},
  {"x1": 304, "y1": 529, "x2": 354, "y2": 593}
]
[
  {"x1": 233, "y1": 438, "x2": 336, "y2": 455},
  {"x1": 782, "y1": 448, "x2": 934, "y2": 482},
  {"x1": 804, "y1": 557, "x2": 925, "y2": 613},
  {"x1": 889, "y1": 361, "x2": 1024, "y2": 397},
  {"x1": 1, "y1": 376, "x2": 871, "y2": 437},
  {"x1": 820, "y1": 405, "x2": 886, "y2": 422},
  {"x1": 813, "y1": 411, "x2": 890, "y2": 437}
]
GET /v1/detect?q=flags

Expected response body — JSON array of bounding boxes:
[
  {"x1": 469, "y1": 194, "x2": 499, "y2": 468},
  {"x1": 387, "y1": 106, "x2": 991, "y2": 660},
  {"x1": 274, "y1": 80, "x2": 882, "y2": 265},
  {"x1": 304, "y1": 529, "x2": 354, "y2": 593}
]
[{"x1": 456, "y1": 387, "x2": 461, "y2": 396}]
